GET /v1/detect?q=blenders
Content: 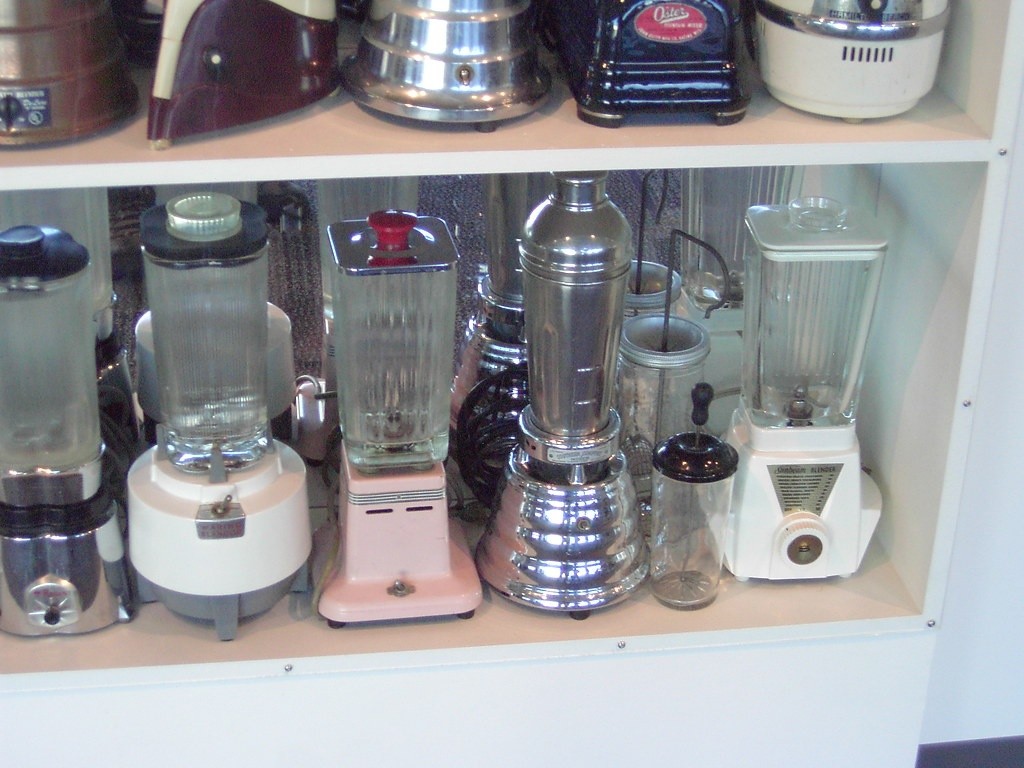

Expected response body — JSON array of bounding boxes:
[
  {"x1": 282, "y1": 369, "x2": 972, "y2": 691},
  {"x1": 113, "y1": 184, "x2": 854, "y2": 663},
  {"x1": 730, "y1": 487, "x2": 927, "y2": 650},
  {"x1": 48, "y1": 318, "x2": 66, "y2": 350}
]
[{"x1": 3, "y1": 1, "x2": 952, "y2": 644}]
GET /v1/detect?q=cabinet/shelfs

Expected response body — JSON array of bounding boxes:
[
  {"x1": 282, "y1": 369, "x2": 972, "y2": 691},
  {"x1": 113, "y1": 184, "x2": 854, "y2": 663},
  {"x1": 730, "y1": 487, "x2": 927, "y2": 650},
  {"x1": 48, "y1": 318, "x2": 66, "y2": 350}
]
[{"x1": 0, "y1": 0, "x2": 1024, "y2": 768}]
